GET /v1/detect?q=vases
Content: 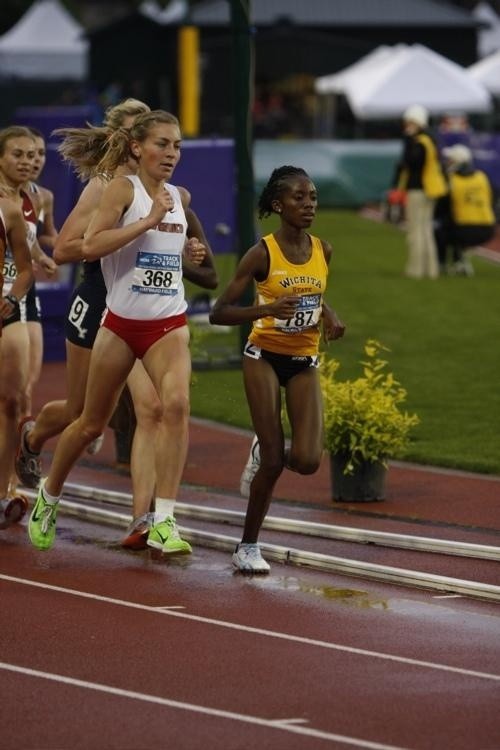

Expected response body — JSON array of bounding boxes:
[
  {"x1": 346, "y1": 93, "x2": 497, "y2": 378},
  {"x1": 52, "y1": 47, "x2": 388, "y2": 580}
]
[{"x1": 329, "y1": 430, "x2": 386, "y2": 502}]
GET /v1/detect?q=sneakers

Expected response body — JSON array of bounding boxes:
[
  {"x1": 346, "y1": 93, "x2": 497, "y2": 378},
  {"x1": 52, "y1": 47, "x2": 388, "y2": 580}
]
[
  {"x1": 28, "y1": 478, "x2": 63, "y2": 552},
  {"x1": 230, "y1": 543, "x2": 271, "y2": 574},
  {"x1": 14, "y1": 416, "x2": 42, "y2": 488},
  {"x1": 147, "y1": 516, "x2": 192, "y2": 555},
  {"x1": 0, "y1": 494, "x2": 28, "y2": 530},
  {"x1": 239, "y1": 434, "x2": 262, "y2": 498},
  {"x1": 123, "y1": 517, "x2": 154, "y2": 552}
]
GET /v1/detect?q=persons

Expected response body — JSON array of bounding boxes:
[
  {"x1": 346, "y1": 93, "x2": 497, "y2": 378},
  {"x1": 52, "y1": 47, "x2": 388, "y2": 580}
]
[
  {"x1": 209, "y1": 165, "x2": 347, "y2": 574},
  {"x1": 0, "y1": 97, "x2": 220, "y2": 555},
  {"x1": 387, "y1": 106, "x2": 496, "y2": 279}
]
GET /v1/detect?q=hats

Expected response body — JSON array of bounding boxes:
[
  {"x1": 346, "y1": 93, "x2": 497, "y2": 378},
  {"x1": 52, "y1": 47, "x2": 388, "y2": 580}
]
[
  {"x1": 403, "y1": 104, "x2": 428, "y2": 132},
  {"x1": 441, "y1": 144, "x2": 473, "y2": 165}
]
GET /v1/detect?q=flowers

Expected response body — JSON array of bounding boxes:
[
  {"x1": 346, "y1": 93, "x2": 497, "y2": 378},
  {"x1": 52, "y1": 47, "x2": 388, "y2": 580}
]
[{"x1": 281, "y1": 336, "x2": 422, "y2": 472}]
